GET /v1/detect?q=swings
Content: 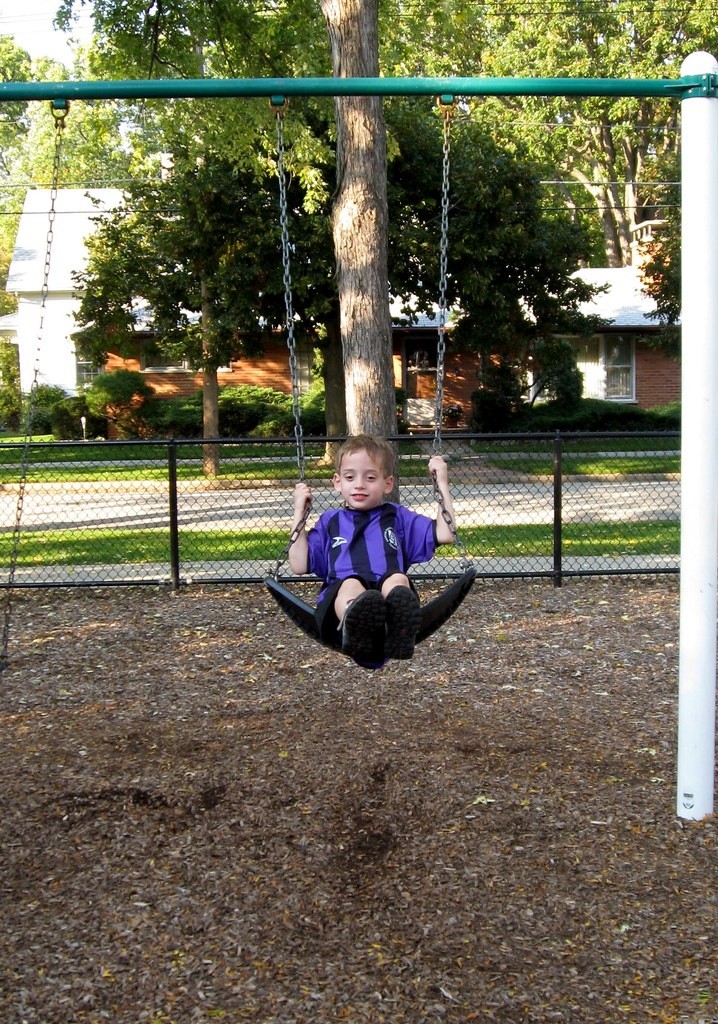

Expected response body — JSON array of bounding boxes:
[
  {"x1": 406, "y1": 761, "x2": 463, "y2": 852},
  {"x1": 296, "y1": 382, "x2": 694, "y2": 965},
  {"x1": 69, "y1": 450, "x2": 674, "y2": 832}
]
[
  {"x1": 263, "y1": 95, "x2": 479, "y2": 658},
  {"x1": 0, "y1": 114, "x2": 68, "y2": 674}
]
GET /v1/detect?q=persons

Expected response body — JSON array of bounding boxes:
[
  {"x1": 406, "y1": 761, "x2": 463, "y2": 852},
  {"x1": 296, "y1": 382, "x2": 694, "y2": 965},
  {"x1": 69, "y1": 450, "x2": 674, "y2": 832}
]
[{"x1": 288, "y1": 434, "x2": 458, "y2": 670}]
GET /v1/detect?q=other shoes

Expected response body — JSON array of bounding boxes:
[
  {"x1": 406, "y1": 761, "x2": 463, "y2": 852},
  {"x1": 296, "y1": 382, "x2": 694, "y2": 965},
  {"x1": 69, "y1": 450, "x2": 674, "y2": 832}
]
[
  {"x1": 337, "y1": 589, "x2": 388, "y2": 670},
  {"x1": 386, "y1": 585, "x2": 422, "y2": 660}
]
